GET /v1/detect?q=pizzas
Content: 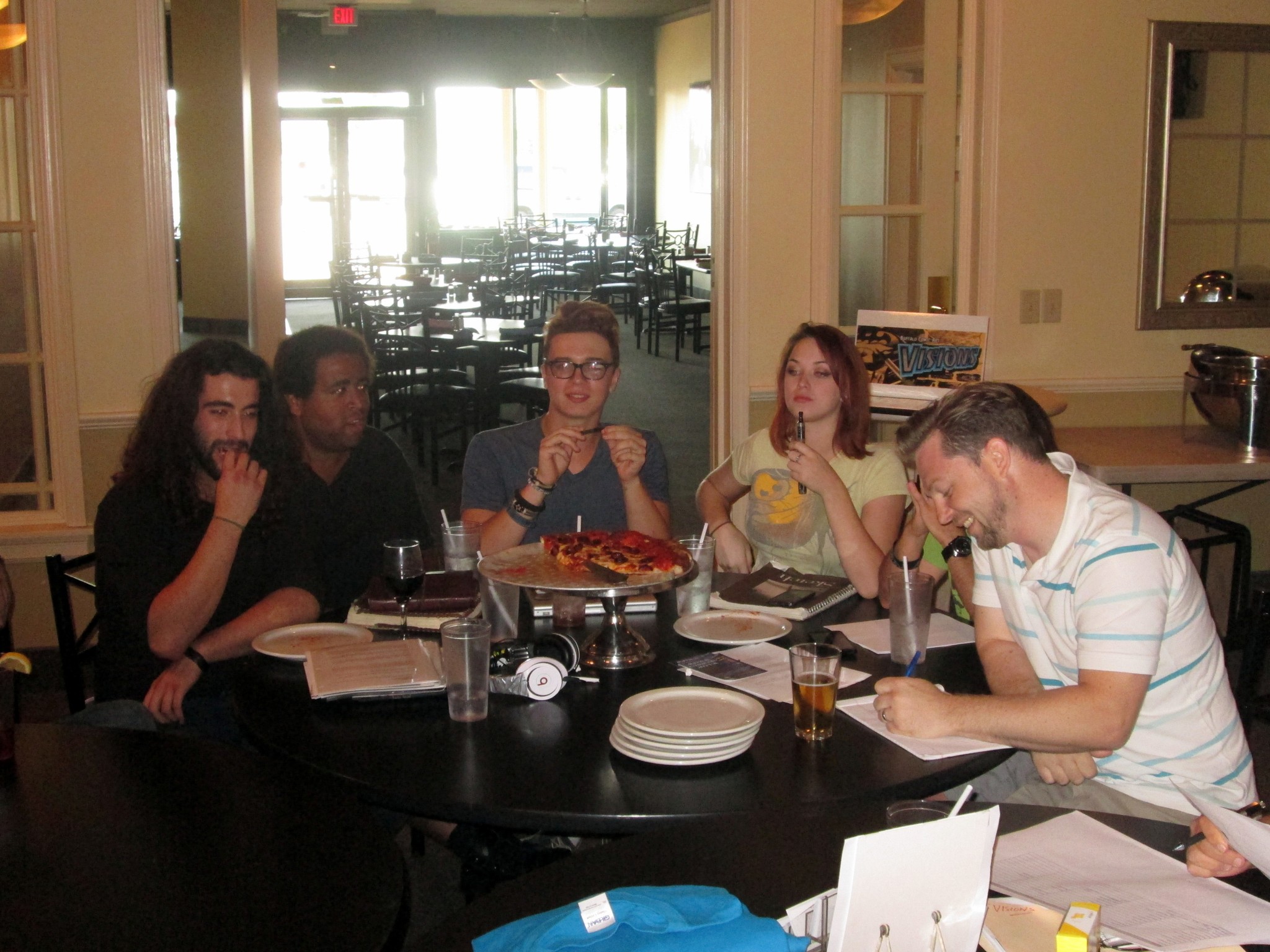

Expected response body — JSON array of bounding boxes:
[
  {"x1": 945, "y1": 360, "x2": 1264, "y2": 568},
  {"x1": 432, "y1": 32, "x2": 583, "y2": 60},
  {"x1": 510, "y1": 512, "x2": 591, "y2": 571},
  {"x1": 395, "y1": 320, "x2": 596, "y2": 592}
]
[{"x1": 540, "y1": 529, "x2": 691, "y2": 575}]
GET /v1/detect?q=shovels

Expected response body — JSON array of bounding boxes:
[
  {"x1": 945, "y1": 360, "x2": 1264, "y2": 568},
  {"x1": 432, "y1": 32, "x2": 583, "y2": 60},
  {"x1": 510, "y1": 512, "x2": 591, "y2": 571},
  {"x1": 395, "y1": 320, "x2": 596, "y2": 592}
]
[{"x1": 581, "y1": 559, "x2": 628, "y2": 584}]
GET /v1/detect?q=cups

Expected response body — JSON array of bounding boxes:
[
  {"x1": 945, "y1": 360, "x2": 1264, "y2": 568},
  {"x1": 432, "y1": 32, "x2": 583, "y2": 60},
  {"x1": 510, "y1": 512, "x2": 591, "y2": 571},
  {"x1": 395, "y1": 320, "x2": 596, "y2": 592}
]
[
  {"x1": 885, "y1": 799, "x2": 955, "y2": 828},
  {"x1": 674, "y1": 534, "x2": 716, "y2": 616},
  {"x1": 885, "y1": 571, "x2": 936, "y2": 664},
  {"x1": 439, "y1": 618, "x2": 491, "y2": 722},
  {"x1": 552, "y1": 592, "x2": 587, "y2": 632},
  {"x1": 440, "y1": 525, "x2": 482, "y2": 577},
  {"x1": 788, "y1": 642, "x2": 842, "y2": 742}
]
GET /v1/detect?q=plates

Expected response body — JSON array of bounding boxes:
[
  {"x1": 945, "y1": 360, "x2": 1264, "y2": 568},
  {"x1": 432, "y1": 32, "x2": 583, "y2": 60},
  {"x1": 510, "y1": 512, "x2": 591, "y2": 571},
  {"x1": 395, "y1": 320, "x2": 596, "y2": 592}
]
[
  {"x1": 251, "y1": 623, "x2": 374, "y2": 661},
  {"x1": 673, "y1": 609, "x2": 794, "y2": 646},
  {"x1": 609, "y1": 686, "x2": 766, "y2": 766}
]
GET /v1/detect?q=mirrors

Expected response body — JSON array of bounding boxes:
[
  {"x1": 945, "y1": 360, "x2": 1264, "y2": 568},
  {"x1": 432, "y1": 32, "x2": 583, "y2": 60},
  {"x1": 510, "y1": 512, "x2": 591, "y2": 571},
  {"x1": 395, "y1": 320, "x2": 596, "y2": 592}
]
[{"x1": 1136, "y1": 18, "x2": 1270, "y2": 330}]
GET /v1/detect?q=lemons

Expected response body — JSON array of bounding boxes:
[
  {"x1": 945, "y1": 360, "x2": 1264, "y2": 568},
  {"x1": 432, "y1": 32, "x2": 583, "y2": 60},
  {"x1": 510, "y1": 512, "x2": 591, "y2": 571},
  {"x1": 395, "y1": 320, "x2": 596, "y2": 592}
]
[{"x1": 0, "y1": 652, "x2": 32, "y2": 674}]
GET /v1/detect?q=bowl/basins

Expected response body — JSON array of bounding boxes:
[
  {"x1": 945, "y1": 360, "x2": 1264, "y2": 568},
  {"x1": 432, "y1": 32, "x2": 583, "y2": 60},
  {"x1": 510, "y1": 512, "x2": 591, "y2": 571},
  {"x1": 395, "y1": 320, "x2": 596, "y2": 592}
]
[{"x1": 1184, "y1": 344, "x2": 1270, "y2": 449}]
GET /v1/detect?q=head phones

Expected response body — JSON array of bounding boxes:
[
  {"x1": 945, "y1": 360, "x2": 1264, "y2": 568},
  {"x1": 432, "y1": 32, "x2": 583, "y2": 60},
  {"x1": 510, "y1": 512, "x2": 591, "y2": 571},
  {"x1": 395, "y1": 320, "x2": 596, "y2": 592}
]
[{"x1": 481, "y1": 630, "x2": 581, "y2": 701}]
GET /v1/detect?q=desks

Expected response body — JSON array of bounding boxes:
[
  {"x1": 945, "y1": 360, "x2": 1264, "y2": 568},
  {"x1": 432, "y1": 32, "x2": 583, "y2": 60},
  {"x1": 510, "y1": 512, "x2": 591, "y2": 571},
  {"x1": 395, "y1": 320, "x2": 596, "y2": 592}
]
[
  {"x1": 354, "y1": 277, "x2": 505, "y2": 292},
  {"x1": 0, "y1": 724, "x2": 411, "y2": 952},
  {"x1": 349, "y1": 257, "x2": 481, "y2": 273},
  {"x1": 363, "y1": 295, "x2": 539, "y2": 321},
  {"x1": 530, "y1": 233, "x2": 646, "y2": 304},
  {"x1": 377, "y1": 317, "x2": 551, "y2": 473},
  {"x1": 1057, "y1": 425, "x2": 1270, "y2": 519},
  {"x1": 401, "y1": 796, "x2": 1270, "y2": 952}
]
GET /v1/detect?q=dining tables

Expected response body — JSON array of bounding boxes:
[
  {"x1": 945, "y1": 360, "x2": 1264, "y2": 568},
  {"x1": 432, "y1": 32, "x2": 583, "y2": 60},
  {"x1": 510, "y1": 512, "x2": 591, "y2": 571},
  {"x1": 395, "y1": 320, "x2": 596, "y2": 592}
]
[{"x1": 181, "y1": 573, "x2": 1018, "y2": 952}]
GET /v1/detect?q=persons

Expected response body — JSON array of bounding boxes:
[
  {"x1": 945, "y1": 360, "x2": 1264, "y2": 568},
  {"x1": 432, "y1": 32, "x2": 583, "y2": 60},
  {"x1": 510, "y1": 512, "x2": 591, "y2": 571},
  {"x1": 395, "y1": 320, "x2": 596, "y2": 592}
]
[
  {"x1": 1186, "y1": 800, "x2": 1270, "y2": 877},
  {"x1": 94, "y1": 338, "x2": 334, "y2": 728},
  {"x1": 873, "y1": 382, "x2": 1259, "y2": 825},
  {"x1": 877, "y1": 383, "x2": 1060, "y2": 620},
  {"x1": 693, "y1": 322, "x2": 912, "y2": 599},
  {"x1": 262, "y1": 324, "x2": 432, "y2": 610},
  {"x1": 457, "y1": 302, "x2": 671, "y2": 561}
]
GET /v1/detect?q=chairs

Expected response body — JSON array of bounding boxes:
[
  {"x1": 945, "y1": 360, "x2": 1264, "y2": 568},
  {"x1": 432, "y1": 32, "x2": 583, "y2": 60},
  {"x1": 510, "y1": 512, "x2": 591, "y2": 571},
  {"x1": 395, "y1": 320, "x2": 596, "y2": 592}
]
[
  {"x1": 329, "y1": 211, "x2": 711, "y2": 489},
  {"x1": 1167, "y1": 504, "x2": 1251, "y2": 741},
  {"x1": 46, "y1": 552, "x2": 98, "y2": 714}
]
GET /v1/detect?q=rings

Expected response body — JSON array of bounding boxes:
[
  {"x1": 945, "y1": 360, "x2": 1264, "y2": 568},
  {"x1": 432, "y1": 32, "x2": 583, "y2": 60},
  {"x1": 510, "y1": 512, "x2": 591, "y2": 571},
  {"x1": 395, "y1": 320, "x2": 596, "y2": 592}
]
[
  {"x1": 881, "y1": 708, "x2": 888, "y2": 722},
  {"x1": 795, "y1": 454, "x2": 802, "y2": 463}
]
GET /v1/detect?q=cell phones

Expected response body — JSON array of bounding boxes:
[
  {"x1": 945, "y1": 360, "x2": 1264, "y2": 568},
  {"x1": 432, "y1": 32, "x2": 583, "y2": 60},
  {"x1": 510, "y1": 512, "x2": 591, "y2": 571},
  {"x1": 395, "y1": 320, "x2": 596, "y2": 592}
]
[{"x1": 809, "y1": 630, "x2": 857, "y2": 656}]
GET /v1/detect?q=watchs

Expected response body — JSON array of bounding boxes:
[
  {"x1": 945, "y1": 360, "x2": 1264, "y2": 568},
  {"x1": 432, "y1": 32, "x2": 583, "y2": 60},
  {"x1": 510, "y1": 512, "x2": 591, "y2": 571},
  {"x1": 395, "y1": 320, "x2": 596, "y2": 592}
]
[{"x1": 941, "y1": 535, "x2": 972, "y2": 562}]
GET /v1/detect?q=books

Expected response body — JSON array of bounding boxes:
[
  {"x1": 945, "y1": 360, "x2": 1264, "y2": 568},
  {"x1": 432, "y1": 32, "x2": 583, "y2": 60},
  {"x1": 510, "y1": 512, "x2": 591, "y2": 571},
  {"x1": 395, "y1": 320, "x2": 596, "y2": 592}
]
[
  {"x1": 833, "y1": 683, "x2": 1015, "y2": 762},
  {"x1": 965, "y1": 806, "x2": 1270, "y2": 951},
  {"x1": 707, "y1": 560, "x2": 854, "y2": 620},
  {"x1": 342, "y1": 547, "x2": 487, "y2": 635}
]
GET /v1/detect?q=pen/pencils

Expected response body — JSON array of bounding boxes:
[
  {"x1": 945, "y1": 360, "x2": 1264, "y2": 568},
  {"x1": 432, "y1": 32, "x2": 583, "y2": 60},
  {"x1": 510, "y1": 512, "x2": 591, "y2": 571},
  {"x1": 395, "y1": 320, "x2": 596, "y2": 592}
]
[
  {"x1": 905, "y1": 651, "x2": 921, "y2": 677},
  {"x1": 1172, "y1": 801, "x2": 1266, "y2": 851},
  {"x1": 797, "y1": 412, "x2": 805, "y2": 494}
]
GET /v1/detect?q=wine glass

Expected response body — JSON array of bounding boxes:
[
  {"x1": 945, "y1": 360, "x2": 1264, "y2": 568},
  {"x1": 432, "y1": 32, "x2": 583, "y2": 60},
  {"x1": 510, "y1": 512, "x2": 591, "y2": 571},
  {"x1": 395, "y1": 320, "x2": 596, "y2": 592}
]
[{"x1": 381, "y1": 538, "x2": 425, "y2": 640}]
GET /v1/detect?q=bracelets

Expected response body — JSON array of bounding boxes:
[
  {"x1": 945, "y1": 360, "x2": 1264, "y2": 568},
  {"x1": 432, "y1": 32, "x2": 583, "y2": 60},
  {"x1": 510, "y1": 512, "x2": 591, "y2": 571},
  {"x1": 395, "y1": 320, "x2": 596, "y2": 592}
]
[
  {"x1": 184, "y1": 646, "x2": 210, "y2": 673},
  {"x1": 527, "y1": 466, "x2": 557, "y2": 497},
  {"x1": 891, "y1": 539, "x2": 924, "y2": 570},
  {"x1": 503, "y1": 488, "x2": 547, "y2": 530},
  {"x1": 707, "y1": 520, "x2": 733, "y2": 537},
  {"x1": 212, "y1": 515, "x2": 246, "y2": 531}
]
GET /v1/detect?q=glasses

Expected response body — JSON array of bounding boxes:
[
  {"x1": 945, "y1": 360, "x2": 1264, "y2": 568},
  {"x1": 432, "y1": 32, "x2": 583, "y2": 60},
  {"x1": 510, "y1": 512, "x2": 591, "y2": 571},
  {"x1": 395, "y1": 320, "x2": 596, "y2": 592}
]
[{"x1": 542, "y1": 358, "x2": 614, "y2": 381}]
